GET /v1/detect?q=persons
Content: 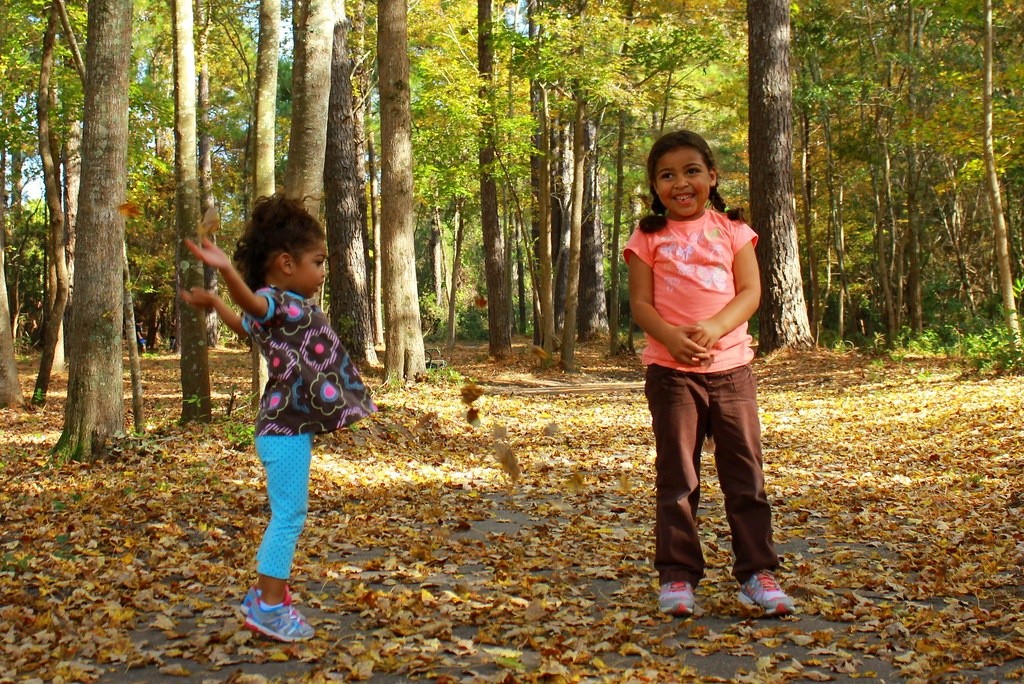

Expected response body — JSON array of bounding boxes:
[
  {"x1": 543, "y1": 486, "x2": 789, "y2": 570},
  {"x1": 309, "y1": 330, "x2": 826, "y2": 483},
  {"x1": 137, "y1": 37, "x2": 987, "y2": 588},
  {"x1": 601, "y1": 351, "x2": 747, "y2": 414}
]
[
  {"x1": 625, "y1": 130, "x2": 796, "y2": 618},
  {"x1": 169, "y1": 331, "x2": 176, "y2": 351},
  {"x1": 177, "y1": 197, "x2": 379, "y2": 640},
  {"x1": 135, "y1": 320, "x2": 147, "y2": 353}
]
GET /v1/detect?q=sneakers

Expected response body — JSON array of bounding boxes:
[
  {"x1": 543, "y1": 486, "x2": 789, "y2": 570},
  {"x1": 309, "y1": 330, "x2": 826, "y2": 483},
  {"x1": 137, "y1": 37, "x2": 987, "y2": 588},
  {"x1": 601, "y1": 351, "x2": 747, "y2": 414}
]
[
  {"x1": 246, "y1": 593, "x2": 315, "y2": 642},
  {"x1": 738, "y1": 569, "x2": 795, "y2": 614},
  {"x1": 239, "y1": 586, "x2": 262, "y2": 617},
  {"x1": 657, "y1": 582, "x2": 694, "y2": 613}
]
